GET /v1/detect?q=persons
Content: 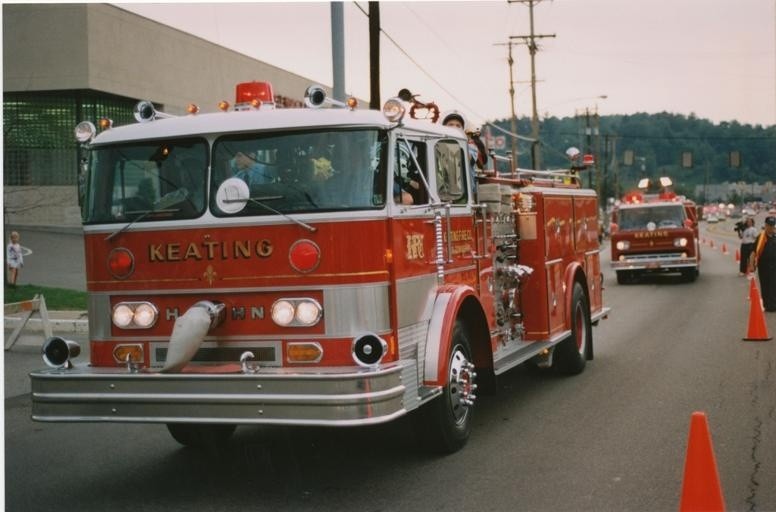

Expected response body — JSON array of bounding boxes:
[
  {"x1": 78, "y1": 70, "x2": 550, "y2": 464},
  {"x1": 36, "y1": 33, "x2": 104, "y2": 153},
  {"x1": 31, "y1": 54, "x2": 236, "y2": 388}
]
[
  {"x1": 233, "y1": 150, "x2": 286, "y2": 184},
  {"x1": 752, "y1": 216, "x2": 776, "y2": 312},
  {"x1": 441, "y1": 112, "x2": 488, "y2": 206},
  {"x1": 322, "y1": 142, "x2": 415, "y2": 204},
  {"x1": 738, "y1": 216, "x2": 756, "y2": 277},
  {"x1": 7, "y1": 230, "x2": 25, "y2": 285}
]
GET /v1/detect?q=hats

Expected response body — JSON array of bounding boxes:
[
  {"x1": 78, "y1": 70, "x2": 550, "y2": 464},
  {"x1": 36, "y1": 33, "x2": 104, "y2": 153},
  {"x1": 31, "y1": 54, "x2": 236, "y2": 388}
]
[{"x1": 765, "y1": 216, "x2": 776, "y2": 226}]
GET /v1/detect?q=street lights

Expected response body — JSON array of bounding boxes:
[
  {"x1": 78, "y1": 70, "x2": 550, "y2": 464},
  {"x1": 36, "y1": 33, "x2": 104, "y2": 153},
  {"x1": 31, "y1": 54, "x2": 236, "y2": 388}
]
[{"x1": 529, "y1": 92, "x2": 608, "y2": 171}]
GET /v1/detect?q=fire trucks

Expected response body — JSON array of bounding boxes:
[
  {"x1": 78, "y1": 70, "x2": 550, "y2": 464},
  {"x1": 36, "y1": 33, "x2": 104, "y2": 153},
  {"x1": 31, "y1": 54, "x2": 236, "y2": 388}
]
[
  {"x1": 611, "y1": 178, "x2": 700, "y2": 284},
  {"x1": 30, "y1": 82, "x2": 610, "y2": 451}
]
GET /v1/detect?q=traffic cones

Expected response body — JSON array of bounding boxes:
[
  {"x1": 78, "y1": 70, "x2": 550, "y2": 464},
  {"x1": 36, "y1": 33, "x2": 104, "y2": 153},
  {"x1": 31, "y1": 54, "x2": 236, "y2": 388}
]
[
  {"x1": 679, "y1": 412, "x2": 726, "y2": 512},
  {"x1": 741, "y1": 277, "x2": 774, "y2": 341},
  {"x1": 703, "y1": 237, "x2": 740, "y2": 261}
]
